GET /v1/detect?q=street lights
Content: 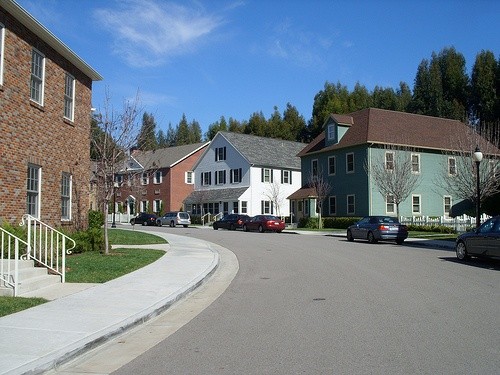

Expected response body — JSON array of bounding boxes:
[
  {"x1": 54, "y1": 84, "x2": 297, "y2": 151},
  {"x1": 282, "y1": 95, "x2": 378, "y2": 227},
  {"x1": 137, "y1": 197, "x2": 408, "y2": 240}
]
[
  {"x1": 473, "y1": 144, "x2": 482, "y2": 231},
  {"x1": 110, "y1": 181, "x2": 119, "y2": 227}
]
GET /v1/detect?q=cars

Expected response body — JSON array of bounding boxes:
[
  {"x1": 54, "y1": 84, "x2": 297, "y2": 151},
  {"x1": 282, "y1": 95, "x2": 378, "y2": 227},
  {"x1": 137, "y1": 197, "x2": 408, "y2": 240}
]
[
  {"x1": 244, "y1": 215, "x2": 285, "y2": 234},
  {"x1": 160, "y1": 210, "x2": 192, "y2": 228},
  {"x1": 456, "y1": 216, "x2": 500, "y2": 260},
  {"x1": 213, "y1": 214, "x2": 251, "y2": 231},
  {"x1": 346, "y1": 215, "x2": 409, "y2": 244},
  {"x1": 129, "y1": 213, "x2": 159, "y2": 225}
]
